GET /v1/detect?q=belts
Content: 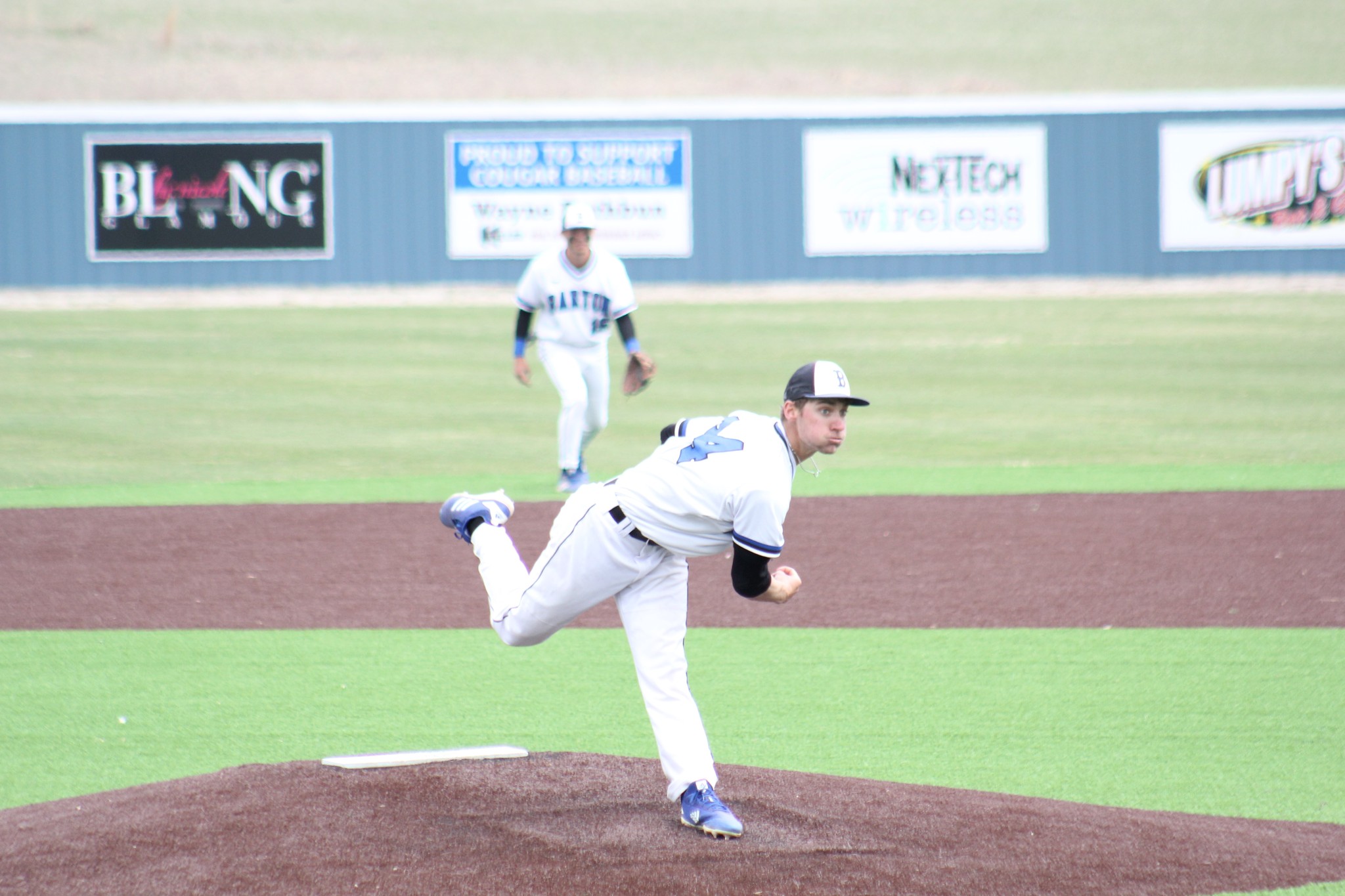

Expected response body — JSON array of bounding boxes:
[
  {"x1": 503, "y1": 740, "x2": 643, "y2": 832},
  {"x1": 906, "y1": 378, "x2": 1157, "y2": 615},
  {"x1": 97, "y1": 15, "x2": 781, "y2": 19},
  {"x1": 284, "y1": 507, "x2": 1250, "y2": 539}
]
[{"x1": 609, "y1": 506, "x2": 657, "y2": 546}]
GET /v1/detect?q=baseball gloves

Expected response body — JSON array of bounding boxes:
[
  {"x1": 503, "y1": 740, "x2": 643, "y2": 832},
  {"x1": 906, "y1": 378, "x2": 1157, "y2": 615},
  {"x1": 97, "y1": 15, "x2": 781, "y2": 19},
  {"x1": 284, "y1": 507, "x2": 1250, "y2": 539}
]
[{"x1": 622, "y1": 354, "x2": 657, "y2": 396}]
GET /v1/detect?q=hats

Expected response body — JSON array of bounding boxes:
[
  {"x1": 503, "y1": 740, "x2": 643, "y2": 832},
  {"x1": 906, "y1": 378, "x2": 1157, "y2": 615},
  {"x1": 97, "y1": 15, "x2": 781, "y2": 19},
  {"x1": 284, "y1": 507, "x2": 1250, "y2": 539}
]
[
  {"x1": 562, "y1": 207, "x2": 594, "y2": 231},
  {"x1": 784, "y1": 361, "x2": 870, "y2": 406}
]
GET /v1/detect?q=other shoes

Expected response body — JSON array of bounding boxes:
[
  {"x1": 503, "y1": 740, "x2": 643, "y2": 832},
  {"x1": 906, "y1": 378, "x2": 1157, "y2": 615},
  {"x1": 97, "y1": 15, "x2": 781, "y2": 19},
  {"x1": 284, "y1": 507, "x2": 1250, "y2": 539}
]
[{"x1": 558, "y1": 470, "x2": 579, "y2": 491}]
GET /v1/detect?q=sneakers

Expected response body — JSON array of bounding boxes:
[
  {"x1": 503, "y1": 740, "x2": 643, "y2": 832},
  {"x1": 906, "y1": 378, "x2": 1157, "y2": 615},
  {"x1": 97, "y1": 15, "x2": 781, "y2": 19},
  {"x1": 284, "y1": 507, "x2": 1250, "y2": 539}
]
[
  {"x1": 439, "y1": 489, "x2": 515, "y2": 543},
  {"x1": 681, "y1": 780, "x2": 742, "y2": 841}
]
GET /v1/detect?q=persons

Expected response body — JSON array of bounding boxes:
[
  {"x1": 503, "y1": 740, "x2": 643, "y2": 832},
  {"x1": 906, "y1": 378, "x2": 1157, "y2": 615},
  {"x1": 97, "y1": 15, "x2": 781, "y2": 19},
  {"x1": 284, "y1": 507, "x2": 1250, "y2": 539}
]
[
  {"x1": 512, "y1": 199, "x2": 641, "y2": 492},
  {"x1": 439, "y1": 360, "x2": 871, "y2": 841}
]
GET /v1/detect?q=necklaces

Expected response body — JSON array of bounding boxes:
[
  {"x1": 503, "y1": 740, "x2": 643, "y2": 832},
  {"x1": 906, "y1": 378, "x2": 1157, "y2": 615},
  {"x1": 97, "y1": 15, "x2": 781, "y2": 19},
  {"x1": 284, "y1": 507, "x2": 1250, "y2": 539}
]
[{"x1": 789, "y1": 442, "x2": 821, "y2": 477}]
